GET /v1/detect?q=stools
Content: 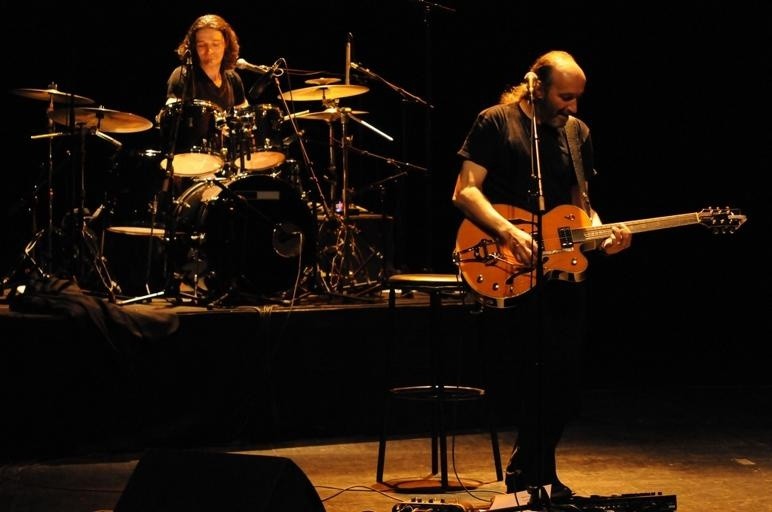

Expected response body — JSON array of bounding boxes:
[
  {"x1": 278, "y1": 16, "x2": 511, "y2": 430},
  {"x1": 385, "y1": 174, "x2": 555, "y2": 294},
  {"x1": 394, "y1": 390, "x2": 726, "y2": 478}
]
[{"x1": 376, "y1": 272, "x2": 505, "y2": 491}]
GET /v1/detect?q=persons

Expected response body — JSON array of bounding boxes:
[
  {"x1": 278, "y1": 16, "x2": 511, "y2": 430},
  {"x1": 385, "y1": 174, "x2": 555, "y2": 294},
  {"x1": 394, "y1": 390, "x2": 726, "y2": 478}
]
[
  {"x1": 161, "y1": 9, "x2": 284, "y2": 306},
  {"x1": 452, "y1": 51, "x2": 632, "y2": 494}
]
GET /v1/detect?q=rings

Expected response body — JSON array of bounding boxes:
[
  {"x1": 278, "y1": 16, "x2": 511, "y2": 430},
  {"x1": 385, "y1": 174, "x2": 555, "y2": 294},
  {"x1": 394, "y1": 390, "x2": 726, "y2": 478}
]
[{"x1": 616, "y1": 242, "x2": 624, "y2": 246}]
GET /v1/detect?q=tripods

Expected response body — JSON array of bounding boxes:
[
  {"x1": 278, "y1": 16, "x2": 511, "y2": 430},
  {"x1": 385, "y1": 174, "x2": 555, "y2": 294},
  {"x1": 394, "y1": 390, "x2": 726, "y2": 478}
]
[
  {"x1": 0, "y1": 118, "x2": 123, "y2": 304},
  {"x1": 293, "y1": 119, "x2": 414, "y2": 302}
]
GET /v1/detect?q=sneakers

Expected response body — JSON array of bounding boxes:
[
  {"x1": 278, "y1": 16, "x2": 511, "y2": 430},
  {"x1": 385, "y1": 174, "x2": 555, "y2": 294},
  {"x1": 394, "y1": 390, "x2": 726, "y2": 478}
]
[{"x1": 507, "y1": 479, "x2": 572, "y2": 498}]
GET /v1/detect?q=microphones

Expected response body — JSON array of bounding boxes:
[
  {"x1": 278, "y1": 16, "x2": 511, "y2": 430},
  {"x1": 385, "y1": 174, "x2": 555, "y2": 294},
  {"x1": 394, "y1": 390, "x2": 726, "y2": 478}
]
[
  {"x1": 185, "y1": 54, "x2": 197, "y2": 100},
  {"x1": 236, "y1": 58, "x2": 270, "y2": 75},
  {"x1": 350, "y1": 61, "x2": 378, "y2": 79},
  {"x1": 524, "y1": 72, "x2": 538, "y2": 93},
  {"x1": 247, "y1": 63, "x2": 280, "y2": 100}
]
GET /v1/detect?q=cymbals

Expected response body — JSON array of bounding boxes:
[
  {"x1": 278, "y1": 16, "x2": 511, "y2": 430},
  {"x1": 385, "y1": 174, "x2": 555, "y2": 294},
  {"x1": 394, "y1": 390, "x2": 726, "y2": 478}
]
[
  {"x1": 277, "y1": 85, "x2": 370, "y2": 101},
  {"x1": 305, "y1": 78, "x2": 341, "y2": 85},
  {"x1": 295, "y1": 108, "x2": 368, "y2": 120},
  {"x1": 47, "y1": 107, "x2": 154, "y2": 133},
  {"x1": 12, "y1": 88, "x2": 94, "y2": 103}
]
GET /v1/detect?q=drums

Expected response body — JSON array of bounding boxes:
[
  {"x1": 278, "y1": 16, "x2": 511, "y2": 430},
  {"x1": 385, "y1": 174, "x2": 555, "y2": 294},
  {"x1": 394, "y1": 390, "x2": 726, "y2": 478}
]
[
  {"x1": 318, "y1": 214, "x2": 394, "y2": 281},
  {"x1": 158, "y1": 99, "x2": 225, "y2": 177},
  {"x1": 172, "y1": 173, "x2": 318, "y2": 306},
  {"x1": 226, "y1": 104, "x2": 286, "y2": 171},
  {"x1": 110, "y1": 226, "x2": 167, "y2": 294}
]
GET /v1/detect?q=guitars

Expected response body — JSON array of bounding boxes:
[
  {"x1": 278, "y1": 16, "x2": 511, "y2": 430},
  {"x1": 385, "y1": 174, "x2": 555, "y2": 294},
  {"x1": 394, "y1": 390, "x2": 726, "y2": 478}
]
[{"x1": 452, "y1": 203, "x2": 748, "y2": 308}]
